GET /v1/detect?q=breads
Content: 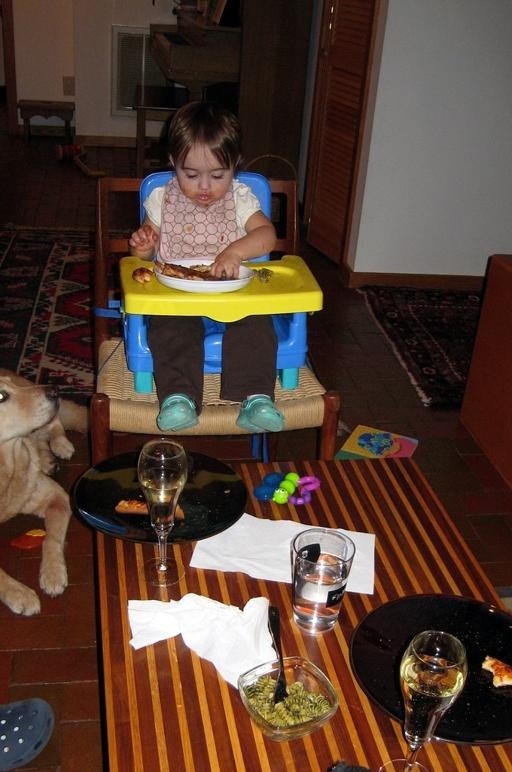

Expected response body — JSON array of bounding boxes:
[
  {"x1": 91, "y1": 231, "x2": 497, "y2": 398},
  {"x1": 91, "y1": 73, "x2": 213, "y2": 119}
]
[
  {"x1": 114, "y1": 499, "x2": 185, "y2": 519},
  {"x1": 133, "y1": 267, "x2": 153, "y2": 284},
  {"x1": 482, "y1": 655, "x2": 511, "y2": 689}
]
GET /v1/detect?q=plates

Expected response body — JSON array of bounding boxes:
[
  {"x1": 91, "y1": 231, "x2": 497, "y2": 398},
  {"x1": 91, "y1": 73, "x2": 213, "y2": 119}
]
[
  {"x1": 71, "y1": 449, "x2": 247, "y2": 545},
  {"x1": 348, "y1": 593, "x2": 512, "y2": 745},
  {"x1": 154, "y1": 259, "x2": 253, "y2": 295}
]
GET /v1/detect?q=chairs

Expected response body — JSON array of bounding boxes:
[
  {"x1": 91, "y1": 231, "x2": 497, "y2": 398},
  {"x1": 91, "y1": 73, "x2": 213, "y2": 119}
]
[
  {"x1": 92, "y1": 174, "x2": 340, "y2": 465},
  {"x1": 125, "y1": 172, "x2": 308, "y2": 394}
]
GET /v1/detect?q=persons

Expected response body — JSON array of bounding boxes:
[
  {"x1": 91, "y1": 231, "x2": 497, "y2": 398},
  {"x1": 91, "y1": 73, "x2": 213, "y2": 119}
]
[{"x1": 127, "y1": 99, "x2": 285, "y2": 436}]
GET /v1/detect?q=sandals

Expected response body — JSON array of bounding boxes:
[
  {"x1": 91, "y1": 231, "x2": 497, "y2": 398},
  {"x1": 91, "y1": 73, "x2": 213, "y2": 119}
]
[
  {"x1": 236, "y1": 394, "x2": 284, "y2": 434},
  {"x1": 154, "y1": 392, "x2": 200, "y2": 432}
]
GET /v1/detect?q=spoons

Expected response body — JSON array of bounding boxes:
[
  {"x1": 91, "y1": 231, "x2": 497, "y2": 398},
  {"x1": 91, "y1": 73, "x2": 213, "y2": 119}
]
[
  {"x1": 266, "y1": 605, "x2": 288, "y2": 703},
  {"x1": 237, "y1": 655, "x2": 341, "y2": 741}
]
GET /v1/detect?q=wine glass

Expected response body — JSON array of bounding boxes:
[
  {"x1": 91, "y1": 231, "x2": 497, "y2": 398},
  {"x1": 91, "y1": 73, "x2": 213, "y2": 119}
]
[
  {"x1": 373, "y1": 631, "x2": 469, "y2": 772},
  {"x1": 135, "y1": 439, "x2": 190, "y2": 586}
]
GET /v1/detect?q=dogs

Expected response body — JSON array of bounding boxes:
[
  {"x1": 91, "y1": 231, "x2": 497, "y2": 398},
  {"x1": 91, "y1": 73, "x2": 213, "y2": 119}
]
[{"x1": 0, "y1": 367, "x2": 130, "y2": 618}]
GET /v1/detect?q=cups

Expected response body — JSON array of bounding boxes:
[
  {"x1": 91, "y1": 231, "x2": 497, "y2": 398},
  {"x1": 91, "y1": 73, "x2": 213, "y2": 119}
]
[{"x1": 289, "y1": 528, "x2": 356, "y2": 635}]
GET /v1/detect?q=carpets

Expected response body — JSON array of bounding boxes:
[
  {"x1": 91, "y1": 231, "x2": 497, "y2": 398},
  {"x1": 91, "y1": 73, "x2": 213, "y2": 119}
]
[
  {"x1": 354, "y1": 284, "x2": 488, "y2": 415},
  {"x1": 0, "y1": 225, "x2": 122, "y2": 409}
]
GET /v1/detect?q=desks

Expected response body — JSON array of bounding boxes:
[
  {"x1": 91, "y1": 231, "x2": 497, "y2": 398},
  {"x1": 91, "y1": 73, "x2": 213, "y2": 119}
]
[{"x1": 117, "y1": 256, "x2": 323, "y2": 324}]
